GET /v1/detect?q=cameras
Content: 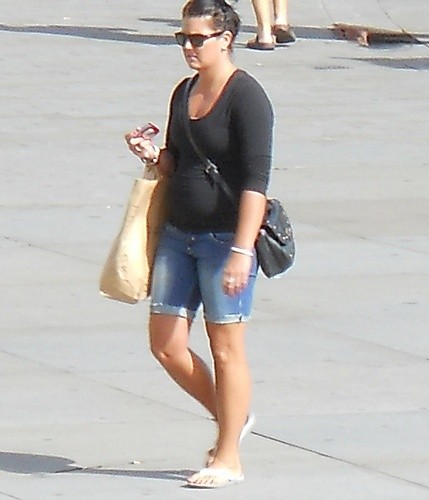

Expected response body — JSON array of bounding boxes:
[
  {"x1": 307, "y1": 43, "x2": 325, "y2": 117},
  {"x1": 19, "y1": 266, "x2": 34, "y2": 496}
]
[{"x1": 135, "y1": 123, "x2": 159, "y2": 140}]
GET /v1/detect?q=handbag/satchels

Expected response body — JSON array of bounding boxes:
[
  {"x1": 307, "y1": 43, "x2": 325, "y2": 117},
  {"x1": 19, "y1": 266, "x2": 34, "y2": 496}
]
[
  {"x1": 255, "y1": 198, "x2": 295, "y2": 278},
  {"x1": 99, "y1": 147, "x2": 167, "y2": 305}
]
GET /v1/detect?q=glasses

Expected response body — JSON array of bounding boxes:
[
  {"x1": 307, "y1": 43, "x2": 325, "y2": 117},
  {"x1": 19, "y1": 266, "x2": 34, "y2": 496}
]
[{"x1": 175, "y1": 31, "x2": 228, "y2": 47}]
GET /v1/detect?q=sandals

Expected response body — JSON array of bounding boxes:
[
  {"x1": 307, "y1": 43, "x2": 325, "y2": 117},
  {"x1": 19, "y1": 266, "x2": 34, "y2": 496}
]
[
  {"x1": 239, "y1": 413, "x2": 255, "y2": 442},
  {"x1": 185, "y1": 468, "x2": 245, "y2": 488},
  {"x1": 248, "y1": 38, "x2": 278, "y2": 50},
  {"x1": 273, "y1": 24, "x2": 295, "y2": 44}
]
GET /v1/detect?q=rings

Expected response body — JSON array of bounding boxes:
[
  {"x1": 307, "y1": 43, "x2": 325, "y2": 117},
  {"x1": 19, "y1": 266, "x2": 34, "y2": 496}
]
[{"x1": 229, "y1": 278, "x2": 235, "y2": 283}]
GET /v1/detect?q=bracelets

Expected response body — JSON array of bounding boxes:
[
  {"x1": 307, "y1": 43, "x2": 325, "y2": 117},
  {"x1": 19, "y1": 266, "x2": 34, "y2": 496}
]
[{"x1": 230, "y1": 246, "x2": 254, "y2": 257}]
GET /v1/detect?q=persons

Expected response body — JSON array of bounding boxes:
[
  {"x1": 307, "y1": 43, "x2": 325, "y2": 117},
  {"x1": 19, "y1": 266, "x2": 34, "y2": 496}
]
[
  {"x1": 247, "y1": 0, "x2": 295, "y2": 50},
  {"x1": 126, "y1": 0, "x2": 274, "y2": 488}
]
